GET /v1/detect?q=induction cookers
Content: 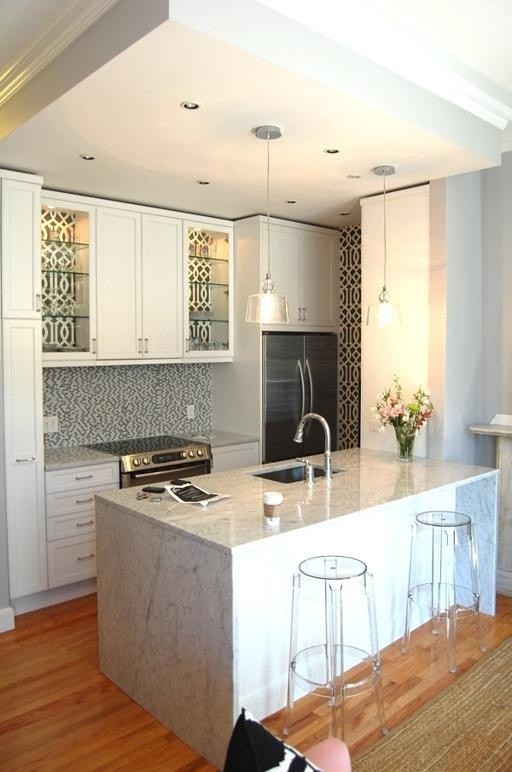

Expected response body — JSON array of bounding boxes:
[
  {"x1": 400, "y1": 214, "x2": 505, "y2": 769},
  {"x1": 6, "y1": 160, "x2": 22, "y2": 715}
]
[{"x1": 80, "y1": 434, "x2": 211, "y2": 485}]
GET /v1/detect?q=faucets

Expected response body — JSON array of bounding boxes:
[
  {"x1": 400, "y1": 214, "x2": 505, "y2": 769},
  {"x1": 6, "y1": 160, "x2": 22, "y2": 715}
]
[{"x1": 293, "y1": 412, "x2": 334, "y2": 481}]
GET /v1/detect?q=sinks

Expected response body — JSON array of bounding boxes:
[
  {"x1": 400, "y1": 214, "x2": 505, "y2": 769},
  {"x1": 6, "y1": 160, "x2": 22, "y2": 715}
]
[{"x1": 246, "y1": 462, "x2": 345, "y2": 484}]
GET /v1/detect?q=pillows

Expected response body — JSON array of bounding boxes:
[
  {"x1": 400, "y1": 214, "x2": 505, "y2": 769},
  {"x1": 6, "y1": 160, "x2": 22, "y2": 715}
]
[{"x1": 221, "y1": 707, "x2": 324, "y2": 772}]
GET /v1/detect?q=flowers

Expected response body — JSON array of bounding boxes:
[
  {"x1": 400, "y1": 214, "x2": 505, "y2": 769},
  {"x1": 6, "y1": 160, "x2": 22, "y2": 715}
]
[{"x1": 370, "y1": 373, "x2": 437, "y2": 459}]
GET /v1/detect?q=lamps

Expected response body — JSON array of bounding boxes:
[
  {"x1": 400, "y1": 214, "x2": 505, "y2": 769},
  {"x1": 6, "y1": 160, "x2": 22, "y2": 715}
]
[
  {"x1": 244, "y1": 125, "x2": 290, "y2": 325},
  {"x1": 366, "y1": 165, "x2": 402, "y2": 328}
]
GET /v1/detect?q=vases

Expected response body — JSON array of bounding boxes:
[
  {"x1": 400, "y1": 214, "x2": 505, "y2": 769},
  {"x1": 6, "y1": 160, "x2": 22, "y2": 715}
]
[{"x1": 394, "y1": 426, "x2": 416, "y2": 462}]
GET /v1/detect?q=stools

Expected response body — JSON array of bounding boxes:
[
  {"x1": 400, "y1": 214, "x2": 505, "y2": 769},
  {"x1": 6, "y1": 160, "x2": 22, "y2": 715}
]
[
  {"x1": 282, "y1": 556, "x2": 389, "y2": 743},
  {"x1": 402, "y1": 509, "x2": 489, "y2": 673}
]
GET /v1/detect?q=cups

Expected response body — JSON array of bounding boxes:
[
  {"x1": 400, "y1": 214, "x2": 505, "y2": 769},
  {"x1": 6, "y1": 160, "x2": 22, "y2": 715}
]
[{"x1": 261, "y1": 492, "x2": 283, "y2": 526}]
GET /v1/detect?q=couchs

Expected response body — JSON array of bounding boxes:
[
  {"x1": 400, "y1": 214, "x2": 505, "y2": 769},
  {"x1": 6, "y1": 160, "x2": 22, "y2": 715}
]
[{"x1": 302, "y1": 738, "x2": 351, "y2": 772}]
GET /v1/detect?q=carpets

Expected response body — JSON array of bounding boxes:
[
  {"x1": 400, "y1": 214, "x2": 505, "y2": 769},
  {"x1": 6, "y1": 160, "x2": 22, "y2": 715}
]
[{"x1": 350, "y1": 634, "x2": 512, "y2": 772}]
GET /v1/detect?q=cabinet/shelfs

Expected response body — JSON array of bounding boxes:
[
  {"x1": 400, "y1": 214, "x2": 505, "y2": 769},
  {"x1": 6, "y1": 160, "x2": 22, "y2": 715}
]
[
  {"x1": 235, "y1": 215, "x2": 338, "y2": 327},
  {"x1": 211, "y1": 442, "x2": 260, "y2": 475},
  {"x1": 1, "y1": 318, "x2": 48, "y2": 602},
  {"x1": 183, "y1": 218, "x2": 234, "y2": 359},
  {"x1": 42, "y1": 196, "x2": 97, "y2": 361},
  {"x1": 0, "y1": 177, "x2": 42, "y2": 319},
  {"x1": 44, "y1": 463, "x2": 122, "y2": 590},
  {"x1": 95, "y1": 205, "x2": 184, "y2": 360}
]
